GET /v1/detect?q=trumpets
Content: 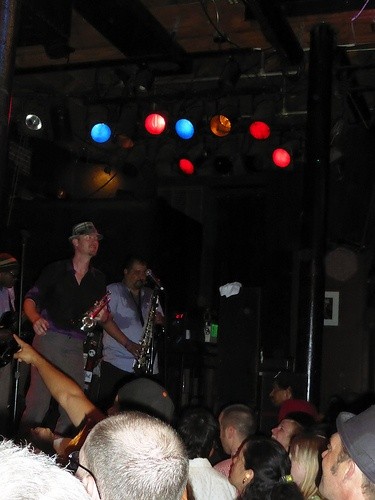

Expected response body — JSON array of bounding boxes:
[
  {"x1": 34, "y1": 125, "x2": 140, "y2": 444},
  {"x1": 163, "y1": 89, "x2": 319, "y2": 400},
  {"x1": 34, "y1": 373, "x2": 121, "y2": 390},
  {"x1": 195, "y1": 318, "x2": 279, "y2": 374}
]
[{"x1": 78, "y1": 289, "x2": 112, "y2": 334}]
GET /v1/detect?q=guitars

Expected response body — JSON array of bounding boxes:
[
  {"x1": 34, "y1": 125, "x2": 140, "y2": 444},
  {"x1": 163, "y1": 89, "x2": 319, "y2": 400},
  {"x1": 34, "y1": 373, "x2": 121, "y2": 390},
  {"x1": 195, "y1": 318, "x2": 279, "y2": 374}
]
[{"x1": 0, "y1": 310, "x2": 36, "y2": 369}]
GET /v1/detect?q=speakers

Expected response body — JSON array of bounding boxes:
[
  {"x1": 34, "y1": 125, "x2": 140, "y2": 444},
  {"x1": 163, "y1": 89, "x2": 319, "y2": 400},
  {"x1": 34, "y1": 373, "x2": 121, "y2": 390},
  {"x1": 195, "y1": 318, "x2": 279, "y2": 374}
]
[{"x1": 214, "y1": 286, "x2": 262, "y2": 411}]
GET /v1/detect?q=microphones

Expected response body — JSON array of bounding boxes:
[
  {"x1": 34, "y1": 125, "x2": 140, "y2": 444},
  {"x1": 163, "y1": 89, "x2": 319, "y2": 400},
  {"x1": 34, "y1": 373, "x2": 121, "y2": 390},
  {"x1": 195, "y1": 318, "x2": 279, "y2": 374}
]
[{"x1": 145, "y1": 269, "x2": 164, "y2": 290}]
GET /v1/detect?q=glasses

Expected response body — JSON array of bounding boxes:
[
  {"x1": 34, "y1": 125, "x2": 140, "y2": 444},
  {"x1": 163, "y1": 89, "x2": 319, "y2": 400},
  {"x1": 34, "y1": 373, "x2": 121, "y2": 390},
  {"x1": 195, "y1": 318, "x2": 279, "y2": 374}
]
[
  {"x1": 8, "y1": 270, "x2": 18, "y2": 278},
  {"x1": 67, "y1": 450, "x2": 102, "y2": 500}
]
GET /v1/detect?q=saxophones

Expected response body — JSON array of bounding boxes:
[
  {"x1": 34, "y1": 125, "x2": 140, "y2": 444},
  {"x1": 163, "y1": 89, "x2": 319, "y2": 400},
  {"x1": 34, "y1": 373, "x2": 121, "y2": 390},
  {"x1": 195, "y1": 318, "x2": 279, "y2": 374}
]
[{"x1": 134, "y1": 282, "x2": 161, "y2": 375}]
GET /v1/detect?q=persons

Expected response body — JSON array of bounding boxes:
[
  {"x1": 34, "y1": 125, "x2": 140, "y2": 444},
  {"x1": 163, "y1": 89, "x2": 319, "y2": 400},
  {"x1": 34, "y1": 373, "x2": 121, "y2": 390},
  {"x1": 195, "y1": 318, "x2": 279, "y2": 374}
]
[
  {"x1": 21, "y1": 222, "x2": 148, "y2": 432},
  {"x1": 0, "y1": 334, "x2": 375, "y2": 500},
  {"x1": 94, "y1": 258, "x2": 159, "y2": 411},
  {"x1": 1, "y1": 251, "x2": 16, "y2": 417}
]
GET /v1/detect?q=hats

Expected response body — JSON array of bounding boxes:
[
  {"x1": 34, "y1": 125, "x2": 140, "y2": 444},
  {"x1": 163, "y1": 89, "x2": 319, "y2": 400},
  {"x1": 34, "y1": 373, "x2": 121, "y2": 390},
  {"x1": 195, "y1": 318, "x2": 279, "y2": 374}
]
[
  {"x1": 0, "y1": 252, "x2": 18, "y2": 268},
  {"x1": 335, "y1": 404, "x2": 375, "y2": 483},
  {"x1": 67, "y1": 221, "x2": 103, "y2": 241},
  {"x1": 118, "y1": 378, "x2": 175, "y2": 425}
]
